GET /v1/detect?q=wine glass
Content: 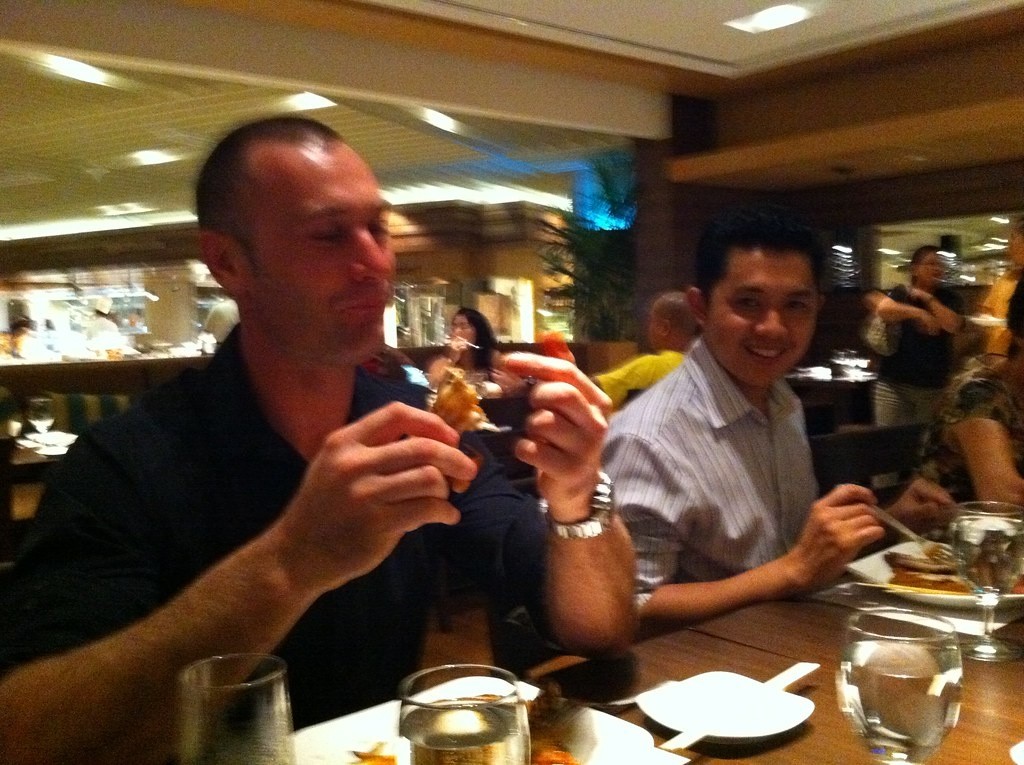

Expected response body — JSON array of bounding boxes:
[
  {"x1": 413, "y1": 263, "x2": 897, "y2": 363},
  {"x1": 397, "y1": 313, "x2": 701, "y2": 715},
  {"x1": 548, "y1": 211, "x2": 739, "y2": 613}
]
[
  {"x1": 836, "y1": 608, "x2": 963, "y2": 765},
  {"x1": 954, "y1": 516, "x2": 1024, "y2": 661}
]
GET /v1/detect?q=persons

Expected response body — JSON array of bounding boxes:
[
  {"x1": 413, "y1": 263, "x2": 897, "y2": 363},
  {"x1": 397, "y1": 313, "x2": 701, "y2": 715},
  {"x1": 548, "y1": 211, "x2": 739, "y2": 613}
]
[
  {"x1": 861, "y1": 245, "x2": 967, "y2": 457},
  {"x1": 426, "y1": 307, "x2": 500, "y2": 392},
  {"x1": 589, "y1": 290, "x2": 696, "y2": 411},
  {"x1": 597, "y1": 204, "x2": 959, "y2": 625},
  {"x1": 204, "y1": 297, "x2": 239, "y2": 352},
  {"x1": 8, "y1": 293, "x2": 119, "y2": 359},
  {"x1": 0, "y1": 113, "x2": 637, "y2": 764},
  {"x1": 885, "y1": 214, "x2": 1024, "y2": 511}
]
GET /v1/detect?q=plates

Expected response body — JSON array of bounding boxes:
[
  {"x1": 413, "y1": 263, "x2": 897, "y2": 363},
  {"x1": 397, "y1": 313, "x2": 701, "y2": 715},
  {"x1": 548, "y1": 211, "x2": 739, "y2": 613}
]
[
  {"x1": 847, "y1": 540, "x2": 1024, "y2": 607},
  {"x1": 293, "y1": 676, "x2": 654, "y2": 765},
  {"x1": 635, "y1": 671, "x2": 815, "y2": 737},
  {"x1": 970, "y1": 317, "x2": 1007, "y2": 327}
]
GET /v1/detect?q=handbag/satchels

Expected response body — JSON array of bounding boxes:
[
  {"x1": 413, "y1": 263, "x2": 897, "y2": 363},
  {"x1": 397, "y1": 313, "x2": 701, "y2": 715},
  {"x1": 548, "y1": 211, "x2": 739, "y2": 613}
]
[{"x1": 860, "y1": 286, "x2": 910, "y2": 355}]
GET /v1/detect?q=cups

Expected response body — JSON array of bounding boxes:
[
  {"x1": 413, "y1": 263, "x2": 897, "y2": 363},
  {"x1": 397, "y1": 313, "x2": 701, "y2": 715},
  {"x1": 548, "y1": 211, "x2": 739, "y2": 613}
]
[
  {"x1": 834, "y1": 349, "x2": 858, "y2": 365},
  {"x1": 399, "y1": 664, "x2": 532, "y2": 765},
  {"x1": 26, "y1": 398, "x2": 57, "y2": 436},
  {"x1": 177, "y1": 653, "x2": 293, "y2": 765},
  {"x1": 958, "y1": 501, "x2": 1024, "y2": 519}
]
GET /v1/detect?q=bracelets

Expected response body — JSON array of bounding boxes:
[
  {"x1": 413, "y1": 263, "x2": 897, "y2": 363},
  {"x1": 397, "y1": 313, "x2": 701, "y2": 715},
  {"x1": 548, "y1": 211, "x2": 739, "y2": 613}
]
[{"x1": 544, "y1": 470, "x2": 615, "y2": 540}]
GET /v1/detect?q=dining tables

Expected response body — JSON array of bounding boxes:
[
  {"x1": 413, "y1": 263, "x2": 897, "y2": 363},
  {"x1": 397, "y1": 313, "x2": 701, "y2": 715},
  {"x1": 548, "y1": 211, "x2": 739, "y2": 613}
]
[{"x1": 283, "y1": 520, "x2": 1024, "y2": 765}]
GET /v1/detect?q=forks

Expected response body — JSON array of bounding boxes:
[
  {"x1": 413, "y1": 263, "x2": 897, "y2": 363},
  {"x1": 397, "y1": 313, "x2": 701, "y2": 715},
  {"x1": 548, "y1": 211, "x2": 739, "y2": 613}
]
[{"x1": 836, "y1": 484, "x2": 966, "y2": 570}]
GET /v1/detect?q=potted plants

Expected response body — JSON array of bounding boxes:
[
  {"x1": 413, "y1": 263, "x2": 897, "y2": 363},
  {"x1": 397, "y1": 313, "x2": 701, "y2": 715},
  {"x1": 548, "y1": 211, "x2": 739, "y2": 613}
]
[{"x1": 531, "y1": 148, "x2": 643, "y2": 370}]
